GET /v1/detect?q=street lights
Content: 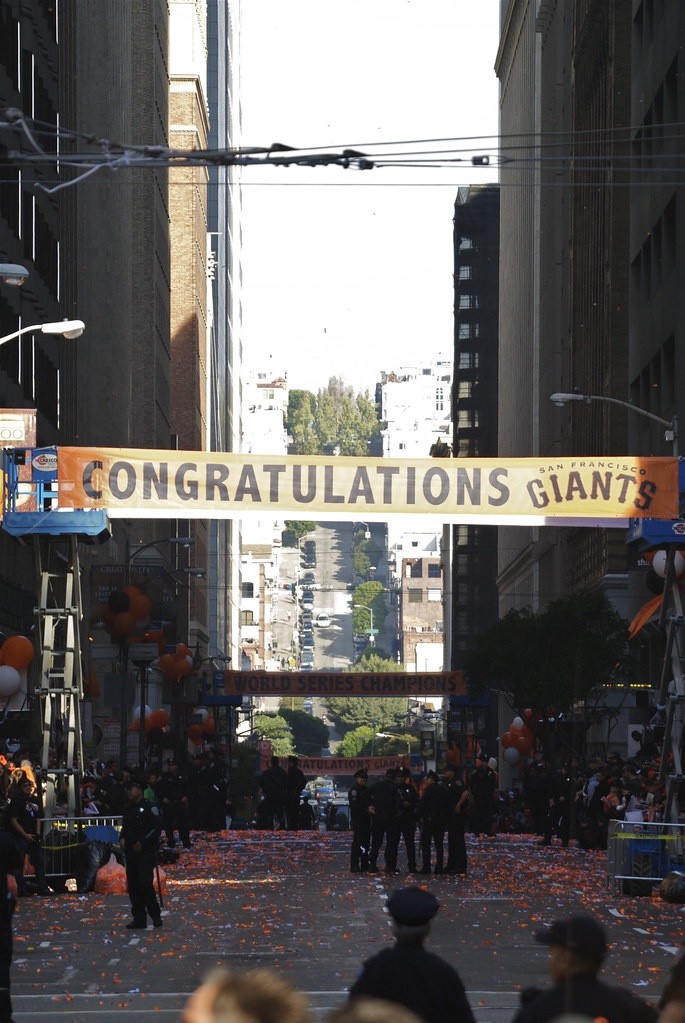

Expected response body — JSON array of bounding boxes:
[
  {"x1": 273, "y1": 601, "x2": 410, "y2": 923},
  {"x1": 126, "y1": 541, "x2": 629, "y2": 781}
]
[
  {"x1": 374, "y1": 734, "x2": 410, "y2": 754},
  {"x1": 293, "y1": 534, "x2": 313, "y2": 634},
  {"x1": 354, "y1": 604, "x2": 372, "y2": 650},
  {"x1": 396, "y1": 713, "x2": 439, "y2": 775},
  {"x1": 226, "y1": 710, "x2": 276, "y2": 802},
  {"x1": 126, "y1": 641, "x2": 160, "y2": 776},
  {"x1": 553, "y1": 392, "x2": 681, "y2": 634},
  {"x1": 116, "y1": 534, "x2": 195, "y2": 769}
]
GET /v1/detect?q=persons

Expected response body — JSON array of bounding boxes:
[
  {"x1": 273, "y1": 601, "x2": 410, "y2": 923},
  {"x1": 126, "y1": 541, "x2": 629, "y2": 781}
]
[
  {"x1": 181, "y1": 885, "x2": 477, "y2": 1023},
  {"x1": 119, "y1": 780, "x2": 163, "y2": 929},
  {"x1": 523, "y1": 730, "x2": 661, "y2": 850},
  {"x1": 255, "y1": 730, "x2": 496, "y2": 875},
  {"x1": 0, "y1": 746, "x2": 230, "y2": 1022},
  {"x1": 512, "y1": 915, "x2": 685, "y2": 1023}
]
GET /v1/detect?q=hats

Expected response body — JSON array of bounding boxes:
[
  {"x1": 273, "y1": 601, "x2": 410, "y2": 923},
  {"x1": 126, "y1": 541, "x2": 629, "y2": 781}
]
[
  {"x1": 395, "y1": 766, "x2": 410, "y2": 777},
  {"x1": 475, "y1": 753, "x2": 490, "y2": 761},
  {"x1": 167, "y1": 758, "x2": 177, "y2": 765},
  {"x1": 534, "y1": 913, "x2": 612, "y2": 959},
  {"x1": 354, "y1": 768, "x2": 368, "y2": 778},
  {"x1": 446, "y1": 763, "x2": 456, "y2": 771},
  {"x1": 387, "y1": 769, "x2": 397, "y2": 777},
  {"x1": 385, "y1": 886, "x2": 440, "y2": 925},
  {"x1": 288, "y1": 755, "x2": 299, "y2": 762},
  {"x1": 124, "y1": 774, "x2": 148, "y2": 790},
  {"x1": 426, "y1": 769, "x2": 439, "y2": 782}
]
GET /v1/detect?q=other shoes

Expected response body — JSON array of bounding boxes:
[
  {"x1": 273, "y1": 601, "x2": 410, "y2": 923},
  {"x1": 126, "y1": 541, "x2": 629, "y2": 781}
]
[
  {"x1": 450, "y1": 867, "x2": 467, "y2": 874},
  {"x1": 384, "y1": 865, "x2": 400, "y2": 872},
  {"x1": 350, "y1": 866, "x2": 361, "y2": 872},
  {"x1": 433, "y1": 867, "x2": 443, "y2": 874},
  {"x1": 368, "y1": 864, "x2": 379, "y2": 872},
  {"x1": 408, "y1": 866, "x2": 417, "y2": 872},
  {"x1": 443, "y1": 866, "x2": 455, "y2": 873},
  {"x1": 37, "y1": 888, "x2": 51, "y2": 895},
  {"x1": 361, "y1": 865, "x2": 369, "y2": 871},
  {"x1": 18, "y1": 891, "x2": 31, "y2": 897},
  {"x1": 126, "y1": 920, "x2": 147, "y2": 928},
  {"x1": 422, "y1": 867, "x2": 432, "y2": 873},
  {"x1": 153, "y1": 918, "x2": 162, "y2": 926}
]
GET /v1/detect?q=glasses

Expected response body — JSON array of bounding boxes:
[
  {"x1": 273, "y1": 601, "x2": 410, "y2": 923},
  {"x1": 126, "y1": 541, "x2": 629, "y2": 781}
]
[{"x1": 21, "y1": 783, "x2": 32, "y2": 787}]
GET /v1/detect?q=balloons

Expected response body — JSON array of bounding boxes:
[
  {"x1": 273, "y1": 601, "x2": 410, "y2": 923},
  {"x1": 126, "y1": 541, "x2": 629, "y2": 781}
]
[
  {"x1": 0, "y1": 665, "x2": 21, "y2": 697},
  {"x1": 500, "y1": 708, "x2": 538, "y2": 765},
  {"x1": 0, "y1": 635, "x2": 34, "y2": 669},
  {"x1": 134, "y1": 704, "x2": 171, "y2": 745},
  {"x1": 653, "y1": 550, "x2": 684, "y2": 577},
  {"x1": 190, "y1": 707, "x2": 215, "y2": 736},
  {"x1": 104, "y1": 584, "x2": 194, "y2": 676}
]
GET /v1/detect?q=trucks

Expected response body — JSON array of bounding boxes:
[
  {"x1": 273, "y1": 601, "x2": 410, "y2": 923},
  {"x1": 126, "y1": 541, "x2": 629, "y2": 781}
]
[{"x1": 314, "y1": 780, "x2": 337, "y2": 803}]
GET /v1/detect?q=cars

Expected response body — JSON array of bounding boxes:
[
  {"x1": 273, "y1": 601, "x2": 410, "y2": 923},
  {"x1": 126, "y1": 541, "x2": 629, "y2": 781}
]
[
  {"x1": 316, "y1": 613, "x2": 332, "y2": 628},
  {"x1": 300, "y1": 572, "x2": 315, "y2": 670},
  {"x1": 353, "y1": 632, "x2": 368, "y2": 664},
  {"x1": 347, "y1": 569, "x2": 365, "y2": 590},
  {"x1": 300, "y1": 786, "x2": 350, "y2": 825},
  {"x1": 321, "y1": 742, "x2": 333, "y2": 757},
  {"x1": 303, "y1": 695, "x2": 314, "y2": 716},
  {"x1": 304, "y1": 540, "x2": 316, "y2": 569}
]
